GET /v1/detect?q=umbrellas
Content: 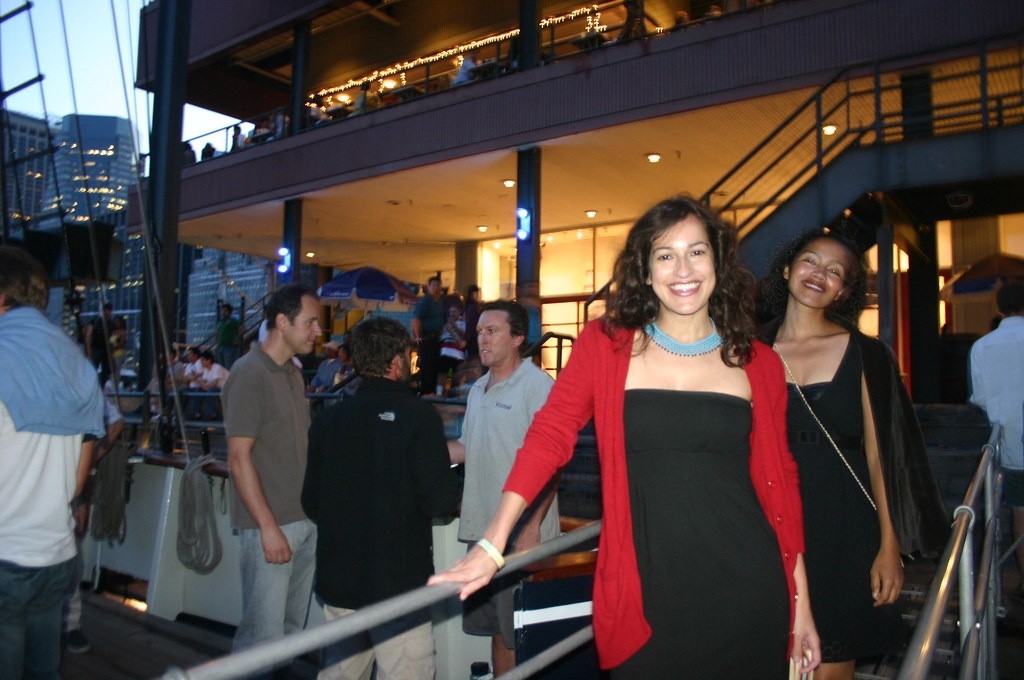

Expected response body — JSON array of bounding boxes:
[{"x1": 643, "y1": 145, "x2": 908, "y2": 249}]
[
  {"x1": 942, "y1": 253, "x2": 1024, "y2": 301},
  {"x1": 317, "y1": 270, "x2": 419, "y2": 319}
]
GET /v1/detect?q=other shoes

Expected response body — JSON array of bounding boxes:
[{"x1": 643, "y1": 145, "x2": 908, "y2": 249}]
[{"x1": 61, "y1": 630, "x2": 91, "y2": 654}]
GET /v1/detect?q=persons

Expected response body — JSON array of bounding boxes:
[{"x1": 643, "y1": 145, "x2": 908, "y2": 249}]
[
  {"x1": 414, "y1": 195, "x2": 821, "y2": 680},
  {"x1": 969, "y1": 280, "x2": 1024, "y2": 578},
  {"x1": 454, "y1": 52, "x2": 479, "y2": 86},
  {"x1": 352, "y1": 81, "x2": 371, "y2": 111},
  {"x1": 1, "y1": 236, "x2": 125, "y2": 680},
  {"x1": 941, "y1": 322, "x2": 953, "y2": 338},
  {"x1": 745, "y1": 228, "x2": 941, "y2": 680},
  {"x1": 183, "y1": 110, "x2": 285, "y2": 164},
  {"x1": 224, "y1": 287, "x2": 451, "y2": 680},
  {"x1": 146, "y1": 302, "x2": 243, "y2": 424}
]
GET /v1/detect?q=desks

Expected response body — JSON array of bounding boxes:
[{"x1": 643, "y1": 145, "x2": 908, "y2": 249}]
[
  {"x1": 470, "y1": 60, "x2": 504, "y2": 80},
  {"x1": 571, "y1": 34, "x2": 616, "y2": 55},
  {"x1": 326, "y1": 106, "x2": 353, "y2": 119},
  {"x1": 250, "y1": 131, "x2": 276, "y2": 143},
  {"x1": 390, "y1": 86, "x2": 426, "y2": 100}
]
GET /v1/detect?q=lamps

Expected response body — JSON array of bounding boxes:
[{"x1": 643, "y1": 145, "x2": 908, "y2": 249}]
[
  {"x1": 648, "y1": 154, "x2": 660, "y2": 163},
  {"x1": 823, "y1": 125, "x2": 838, "y2": 135},
  {"x1": 586, "y1": 210, "x2": 598, "y2": 217},
  {"x1": 478, "y1": 226, "x2": 488, "y2": 233},
  {"x1": 504, "y1": 180, "x2": 515, "y2": 187}
]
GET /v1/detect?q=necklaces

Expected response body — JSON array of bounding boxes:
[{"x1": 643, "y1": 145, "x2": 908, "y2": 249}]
[{"x1": 642, "y1": 316, "x2": 725, "y2": 357}]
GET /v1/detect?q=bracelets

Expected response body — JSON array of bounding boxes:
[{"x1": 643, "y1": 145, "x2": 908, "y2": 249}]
[{"x1": 476, "y1": 538, "x2": 506, "y2": 570}]
